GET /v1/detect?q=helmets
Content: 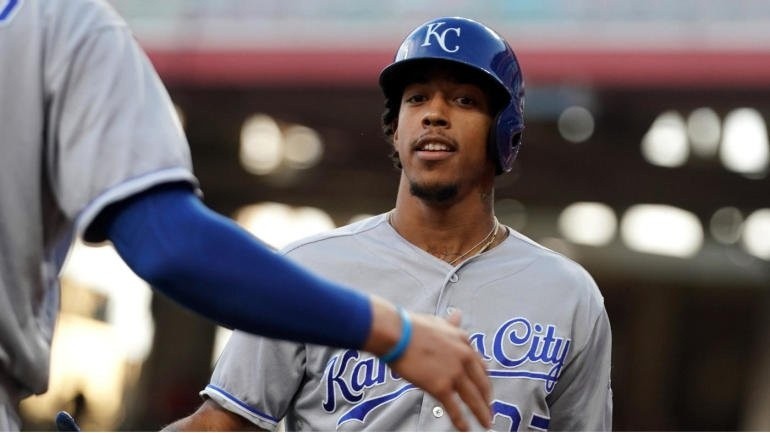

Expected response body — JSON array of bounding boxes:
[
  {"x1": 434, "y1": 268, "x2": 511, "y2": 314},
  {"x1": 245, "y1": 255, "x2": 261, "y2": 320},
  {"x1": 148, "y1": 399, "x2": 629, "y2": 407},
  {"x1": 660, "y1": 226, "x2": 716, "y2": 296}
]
[{"x1": 379, "y1": 17, "x2": 525, "y2": 173}]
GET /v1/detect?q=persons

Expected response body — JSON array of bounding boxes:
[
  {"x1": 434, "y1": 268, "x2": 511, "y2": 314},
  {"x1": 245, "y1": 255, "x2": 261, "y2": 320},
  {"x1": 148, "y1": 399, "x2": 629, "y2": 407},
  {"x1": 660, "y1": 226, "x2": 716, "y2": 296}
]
[
  {"x1": 159, "y1": 16, "x2": 612, "y2": 432},
  {"x1": 0, "y1": 1, "x2": 495, "y2": 432}
]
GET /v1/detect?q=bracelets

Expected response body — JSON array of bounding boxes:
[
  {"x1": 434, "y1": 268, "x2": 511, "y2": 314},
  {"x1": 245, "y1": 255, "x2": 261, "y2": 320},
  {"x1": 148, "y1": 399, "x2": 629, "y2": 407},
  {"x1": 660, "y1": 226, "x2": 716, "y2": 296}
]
[{"x1": 377, "y1": 306, "x2": 413, "y2": 364}]
